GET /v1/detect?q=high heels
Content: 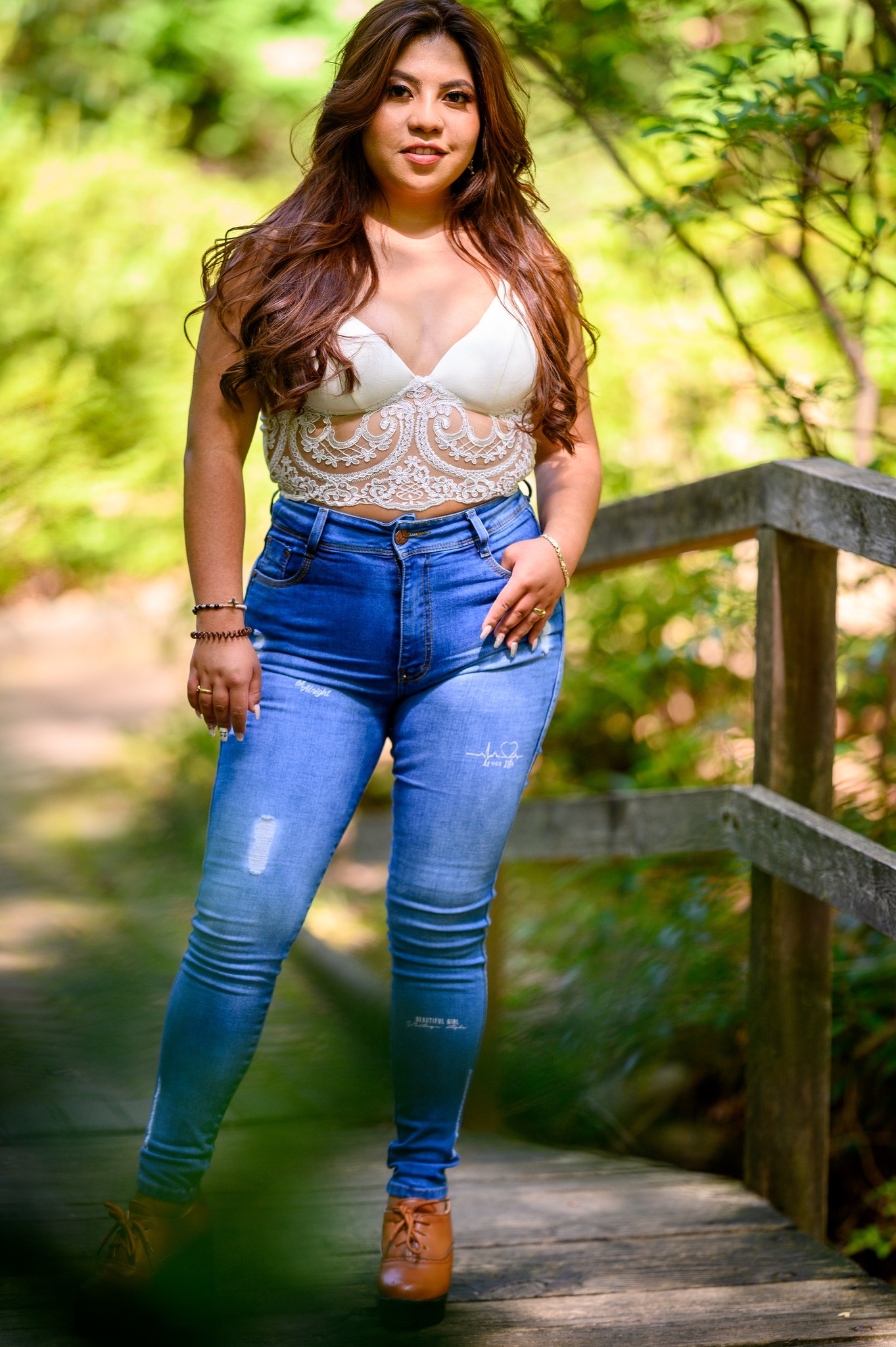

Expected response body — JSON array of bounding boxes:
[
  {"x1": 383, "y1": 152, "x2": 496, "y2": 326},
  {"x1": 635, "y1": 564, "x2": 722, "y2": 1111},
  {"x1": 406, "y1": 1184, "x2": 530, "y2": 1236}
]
[
  {"x1": 374, "y1": 1195, "x2": 454, "y2": 1319},
  {"x1": 77, "y1": 1191, "x2": 211, "y2": 1289}
]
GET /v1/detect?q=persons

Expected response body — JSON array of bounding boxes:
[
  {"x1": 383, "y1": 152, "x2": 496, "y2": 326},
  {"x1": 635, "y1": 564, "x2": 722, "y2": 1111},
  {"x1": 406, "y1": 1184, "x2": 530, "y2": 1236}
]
[{"x1": 84, "y1": 0, "x2": 606, "y2": 1329}]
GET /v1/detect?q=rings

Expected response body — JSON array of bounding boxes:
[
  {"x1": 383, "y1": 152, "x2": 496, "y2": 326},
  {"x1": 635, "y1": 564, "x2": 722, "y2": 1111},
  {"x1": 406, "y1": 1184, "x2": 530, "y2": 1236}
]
[{"x1": 532, "y1": 607, "x2": 546, "y2": 618}]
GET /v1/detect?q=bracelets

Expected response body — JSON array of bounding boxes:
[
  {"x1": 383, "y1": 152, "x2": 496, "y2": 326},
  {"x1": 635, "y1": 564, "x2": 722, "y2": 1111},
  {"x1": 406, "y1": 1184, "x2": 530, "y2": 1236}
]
[
  {"x1": 192, "y1": 596, "x2": 247, "y2": 615},
  {"x1": 191, "y1": 626, "x2": 252, "y2": 639},
  {"x1": 539, "y1": 534, "x2": 570, "y2": 588}
]
